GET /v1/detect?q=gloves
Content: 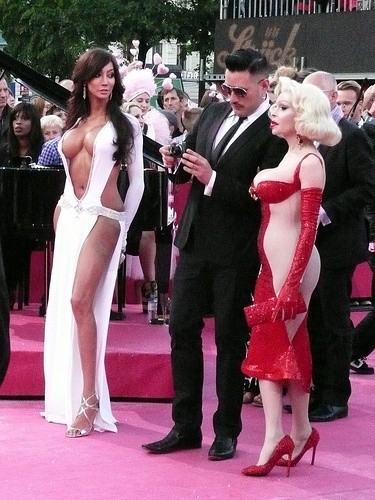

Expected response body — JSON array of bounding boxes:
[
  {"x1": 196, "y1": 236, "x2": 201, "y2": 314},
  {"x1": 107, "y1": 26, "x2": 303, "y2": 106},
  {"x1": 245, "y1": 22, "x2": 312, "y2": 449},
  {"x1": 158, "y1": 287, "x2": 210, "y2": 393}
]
[{"x1": 271, "y1": 188, "x2": 322, "y2": 322}]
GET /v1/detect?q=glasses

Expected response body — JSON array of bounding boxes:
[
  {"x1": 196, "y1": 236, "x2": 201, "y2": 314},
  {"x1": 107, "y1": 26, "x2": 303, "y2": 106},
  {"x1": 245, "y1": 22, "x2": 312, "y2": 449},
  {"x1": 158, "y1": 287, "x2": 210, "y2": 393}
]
[{"x1": 220, "y1": 79, "x2": 264, "y2": 97}]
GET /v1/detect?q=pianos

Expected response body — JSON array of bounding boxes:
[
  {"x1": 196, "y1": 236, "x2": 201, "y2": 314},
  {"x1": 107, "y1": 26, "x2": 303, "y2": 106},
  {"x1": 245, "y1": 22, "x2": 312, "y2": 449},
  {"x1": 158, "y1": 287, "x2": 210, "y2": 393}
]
[{"x1": 0, "y1": 50, "x2": 177, "y2": 321}]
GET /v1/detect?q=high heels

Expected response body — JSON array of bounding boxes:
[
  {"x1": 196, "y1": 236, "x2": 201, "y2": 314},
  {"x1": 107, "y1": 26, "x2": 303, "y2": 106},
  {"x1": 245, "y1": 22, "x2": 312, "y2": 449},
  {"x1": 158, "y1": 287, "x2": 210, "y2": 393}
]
[
  {"x1": 240, "y1": 434, "x2": 295, "y2": 477},
  {"x1": 276, "y1": 427, "x2": 320, "y2": 466},
  {"x1": 142, "y1": 281, "x2": 171, "y2": 314},
  {"x1": 65, "y1": 393, "x2": 99, "y2": 438}
]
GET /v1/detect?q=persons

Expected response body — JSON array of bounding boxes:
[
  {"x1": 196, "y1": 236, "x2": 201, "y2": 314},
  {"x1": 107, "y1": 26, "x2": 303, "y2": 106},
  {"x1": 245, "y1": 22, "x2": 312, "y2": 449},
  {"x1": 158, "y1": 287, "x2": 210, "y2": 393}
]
[
  {"x1": 0, "y1": 62, "x2": 375, "y2": 407},
  {"x1": 139, "y1": 47, "x2": 289, "y2": 460},
  {"x1": 283, "y1": 69, "x2": 375, "y2": 422},
  {"x1": 41, "y1": 48, "x2": 143, "y2": 439},
  {"x1": 242, "y1": 77, "x2": 342, "y2": 478}
]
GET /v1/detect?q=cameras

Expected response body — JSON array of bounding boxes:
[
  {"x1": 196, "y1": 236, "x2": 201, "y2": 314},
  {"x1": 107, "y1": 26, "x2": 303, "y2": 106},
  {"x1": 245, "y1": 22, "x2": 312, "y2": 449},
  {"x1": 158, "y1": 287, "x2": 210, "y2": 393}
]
[{"x1": 171, "y1": 141, "x2": 187, "y2": 157}]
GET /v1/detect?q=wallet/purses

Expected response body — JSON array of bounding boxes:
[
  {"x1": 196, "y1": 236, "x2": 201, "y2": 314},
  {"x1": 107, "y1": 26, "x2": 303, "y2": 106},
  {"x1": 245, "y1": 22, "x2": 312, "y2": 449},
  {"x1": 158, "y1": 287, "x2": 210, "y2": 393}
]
[{"x1": 243, "y1": 292, "x2": 306, "y2": 327}]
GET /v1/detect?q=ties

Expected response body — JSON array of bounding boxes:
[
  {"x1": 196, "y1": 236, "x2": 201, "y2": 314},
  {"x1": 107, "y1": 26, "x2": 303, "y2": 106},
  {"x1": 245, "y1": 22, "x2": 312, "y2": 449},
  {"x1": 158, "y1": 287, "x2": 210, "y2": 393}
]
[{"x1": 211, "y1": 117, "x2": 247, "y2": 165}]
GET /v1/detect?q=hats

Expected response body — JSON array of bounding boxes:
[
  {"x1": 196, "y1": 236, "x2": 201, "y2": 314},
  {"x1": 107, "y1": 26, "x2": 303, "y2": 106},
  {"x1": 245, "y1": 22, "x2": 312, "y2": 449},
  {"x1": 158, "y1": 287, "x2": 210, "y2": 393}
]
[{"x1": 112, "y1": 41, "x2": 177, "y2": 102}]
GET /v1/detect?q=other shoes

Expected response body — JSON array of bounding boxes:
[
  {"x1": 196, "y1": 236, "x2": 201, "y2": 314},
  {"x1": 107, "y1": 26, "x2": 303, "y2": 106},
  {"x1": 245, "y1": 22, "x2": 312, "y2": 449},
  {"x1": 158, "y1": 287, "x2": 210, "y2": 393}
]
[
  {"x1": 349, "y1": 356, "x2": 374, "y2": 375},
  {"x1": 252, "y1": 394, "x2": 263, "y2": 407},
  {"x1": 243, "y1": 392, "x2": 254, "y2": 403},
  {"x1": 110, "y1": 310, "x2": 126, "y2": 320}
]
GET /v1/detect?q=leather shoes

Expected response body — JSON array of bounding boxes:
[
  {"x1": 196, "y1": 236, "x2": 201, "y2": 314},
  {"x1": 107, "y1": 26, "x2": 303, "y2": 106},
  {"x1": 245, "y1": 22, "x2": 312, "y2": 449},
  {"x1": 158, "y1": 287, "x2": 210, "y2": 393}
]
[
  {"x1": 208, "y1": 435, "x2": 239, "y2": 459},
  {"x1": 283, "y1": 400, "x2": 314, "y2": 413},
  {"x1": 308, "y1": 403, "x2": 348, "y2": 421},
  {"x1": 142, "y1": 428, "x2": 203, "y2": 453}
]
